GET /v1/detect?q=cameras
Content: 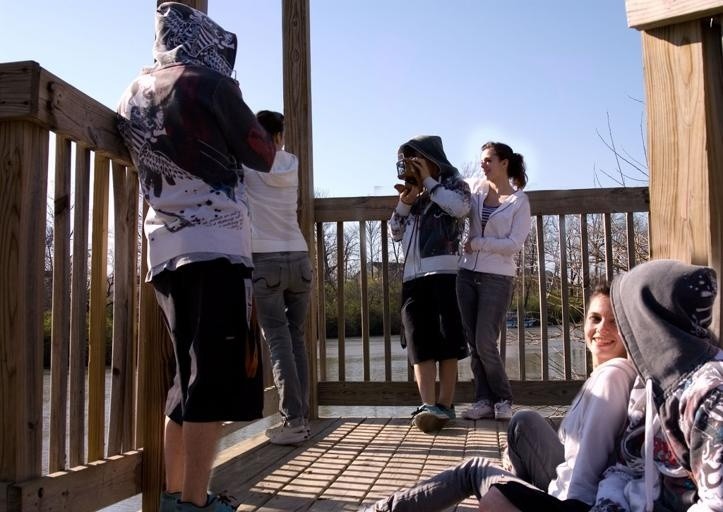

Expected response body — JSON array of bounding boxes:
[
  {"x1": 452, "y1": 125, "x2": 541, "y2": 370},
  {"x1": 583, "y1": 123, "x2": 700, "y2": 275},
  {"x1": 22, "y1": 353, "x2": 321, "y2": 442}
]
[{"x1": 396, "y1": 159, "x2": 421, "y2": 191}]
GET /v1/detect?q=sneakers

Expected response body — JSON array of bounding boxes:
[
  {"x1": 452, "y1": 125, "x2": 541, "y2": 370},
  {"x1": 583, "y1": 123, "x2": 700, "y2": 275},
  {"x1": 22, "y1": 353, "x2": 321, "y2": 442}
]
[
  {"x1": 410, "y1": 404, "x2": 457, "y2": 432},
  {"x1": 459, "y1": 399, "x2": 494, "y2": 420},
  {"x1": 159, "y1": 489, "x2": 239, "y2": 511},
  {"x1": 493, "y1": 397, "x2": 512, "y2": 420},
  {"x1": 265, "y1": 423, "x2": 311, "y2": 445}
]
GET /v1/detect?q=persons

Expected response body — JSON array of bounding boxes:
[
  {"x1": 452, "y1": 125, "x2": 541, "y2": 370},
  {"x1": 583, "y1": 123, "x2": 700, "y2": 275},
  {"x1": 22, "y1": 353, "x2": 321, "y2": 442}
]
[
  {"x1": 388, "y1": 134, "x2": 478, "y2": 433},
  {"x1": 350, "y1": 277, "x2": 639, "y2": 512},
  {"x1": 240, "y1": 108, "x2": 321, "y2": 446},
  {"x1": 119, "y1": 0, "x2": 279, "y2": 512},
  {"x1": 456, "y1": 138, "x2": 535, "y2": 424},
  {"x1": 474, "y1": 256, "x2": 723, "y2": 512}
]
